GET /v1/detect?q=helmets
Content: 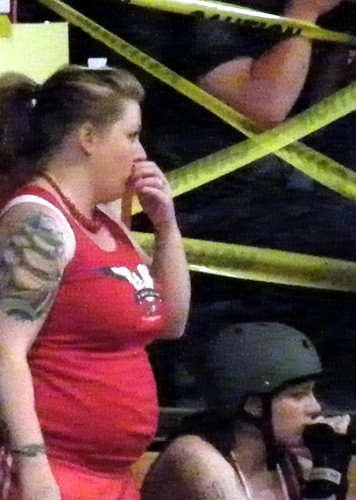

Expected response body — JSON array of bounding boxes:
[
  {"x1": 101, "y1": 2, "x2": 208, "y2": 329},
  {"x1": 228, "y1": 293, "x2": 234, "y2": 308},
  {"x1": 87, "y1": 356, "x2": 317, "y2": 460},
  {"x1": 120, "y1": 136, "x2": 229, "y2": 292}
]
[{"x1": 195, "y1": 323, "x2": 323, "y2": 424}]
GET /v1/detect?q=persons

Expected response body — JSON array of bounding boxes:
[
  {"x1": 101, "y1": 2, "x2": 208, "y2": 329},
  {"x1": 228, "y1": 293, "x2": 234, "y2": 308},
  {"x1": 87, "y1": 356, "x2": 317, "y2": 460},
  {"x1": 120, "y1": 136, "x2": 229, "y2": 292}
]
[
  {"x1": 141, "y1": 323, "x2": 352, "y2": 500},
  {"x1": 0, "y1": 69, "x2": 190, "y2": 500},
  {"x1": 192, "y1": 1, "x2": 341, "y2": 124}
]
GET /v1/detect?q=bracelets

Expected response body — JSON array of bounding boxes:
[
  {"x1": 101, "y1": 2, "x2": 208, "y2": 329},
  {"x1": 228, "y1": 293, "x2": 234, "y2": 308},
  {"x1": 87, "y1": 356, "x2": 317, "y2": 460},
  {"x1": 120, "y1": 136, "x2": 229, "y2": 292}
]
[{"x1": 7, "y1": 448, "x2": 42, "y2": 456}]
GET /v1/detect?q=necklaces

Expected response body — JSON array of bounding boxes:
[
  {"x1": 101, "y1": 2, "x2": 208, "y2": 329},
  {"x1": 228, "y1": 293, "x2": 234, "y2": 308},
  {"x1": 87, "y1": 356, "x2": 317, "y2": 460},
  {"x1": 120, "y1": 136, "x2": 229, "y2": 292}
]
[{"x1": 39, "y1": 172, "x2": 100, "y2": 232}]
